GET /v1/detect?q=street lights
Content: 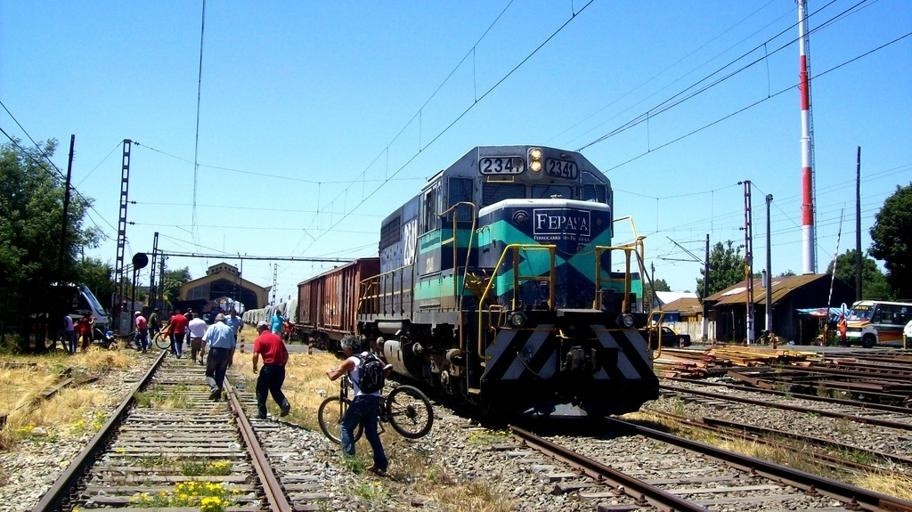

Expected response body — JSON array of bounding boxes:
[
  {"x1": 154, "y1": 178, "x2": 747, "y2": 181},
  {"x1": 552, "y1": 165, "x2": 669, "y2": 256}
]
[{"x1": 762, "y1": 193, "x2": 774, "y2": 338}]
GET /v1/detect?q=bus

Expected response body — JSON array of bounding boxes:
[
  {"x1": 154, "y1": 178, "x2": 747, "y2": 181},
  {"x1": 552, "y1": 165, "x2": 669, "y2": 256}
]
[
  {"x1": 31, "y1": 281, "x2": 113, "y2": 340},
  {"x1": 836, "y1": 300, "x2": 912, "y2": 348}
]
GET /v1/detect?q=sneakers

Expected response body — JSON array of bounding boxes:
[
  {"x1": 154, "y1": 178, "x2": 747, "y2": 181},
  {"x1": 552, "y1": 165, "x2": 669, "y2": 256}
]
[
  {"x1": 254, "y1": 414, "x2": 266, "y2": 419},
  {"x1": 209, "y1": 390, "x2": 221, "y2": 400},
  {"x1": 215, "y1": 397, "x2": 221, "y2": 402},
  {"x1": 281, "y1": 402, "x2": 290, "y2": 417}
]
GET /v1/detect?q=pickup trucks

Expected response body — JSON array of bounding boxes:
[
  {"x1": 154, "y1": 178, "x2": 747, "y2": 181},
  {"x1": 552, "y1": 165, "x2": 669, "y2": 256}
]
[{"x1": 645, "y1": 326, "x2": 692, "y2": 349}]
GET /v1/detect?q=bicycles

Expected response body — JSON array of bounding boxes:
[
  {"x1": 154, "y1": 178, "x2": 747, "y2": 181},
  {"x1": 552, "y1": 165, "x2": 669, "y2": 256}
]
[
  {"x1": 127, "y1": 325, "x2": 172, "y2": 350},
  {"x1": 318, "y1": 364, "x2": 433, "y2": 446}
]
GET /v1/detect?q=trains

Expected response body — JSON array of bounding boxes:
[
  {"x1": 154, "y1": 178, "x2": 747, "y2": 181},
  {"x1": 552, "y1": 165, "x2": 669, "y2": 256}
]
[{"x1": 241, "y1": 144, "x2": 664, "y2": 427}]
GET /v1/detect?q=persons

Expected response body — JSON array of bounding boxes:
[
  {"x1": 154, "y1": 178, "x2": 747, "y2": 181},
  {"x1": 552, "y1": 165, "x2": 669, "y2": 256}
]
[
  {"x1": 269, "y1": 309, "x2": 284, "y2": 336},
  {"x1": 200, "y1": 312, "x2": 236, "y2": 402},
  {"x1": 325, "y1": 333, "x2": 389, "y2": 475},
  {"x1": 251, "y1": 321, "x2": 291, "y2": 419},
  {"x1": 836, "y1": 314, "x2": 847, "y2": 345},
  {"x1": 281, "y1": 316, "x2": 295, "y2": 344},
  {"x1": 47, "y1": 306, "x2": 244, "y2": 368}
]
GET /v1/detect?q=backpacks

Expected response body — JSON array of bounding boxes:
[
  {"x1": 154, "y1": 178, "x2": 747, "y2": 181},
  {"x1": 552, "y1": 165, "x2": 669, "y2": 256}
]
[{"x1": 347, "y1": 351, "x2": 385, "y2": 395}]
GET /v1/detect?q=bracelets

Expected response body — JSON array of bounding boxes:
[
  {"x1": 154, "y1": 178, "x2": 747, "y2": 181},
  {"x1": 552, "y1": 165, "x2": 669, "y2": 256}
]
[{"x1": 253, "y1": 365, "x2": 258, "y2": 369}]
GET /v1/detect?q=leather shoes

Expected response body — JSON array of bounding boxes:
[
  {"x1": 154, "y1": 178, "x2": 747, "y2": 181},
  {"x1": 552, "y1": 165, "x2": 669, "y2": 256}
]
[{"x1": 365, "y1": 463, "x2": 386, "y2": 473}]
